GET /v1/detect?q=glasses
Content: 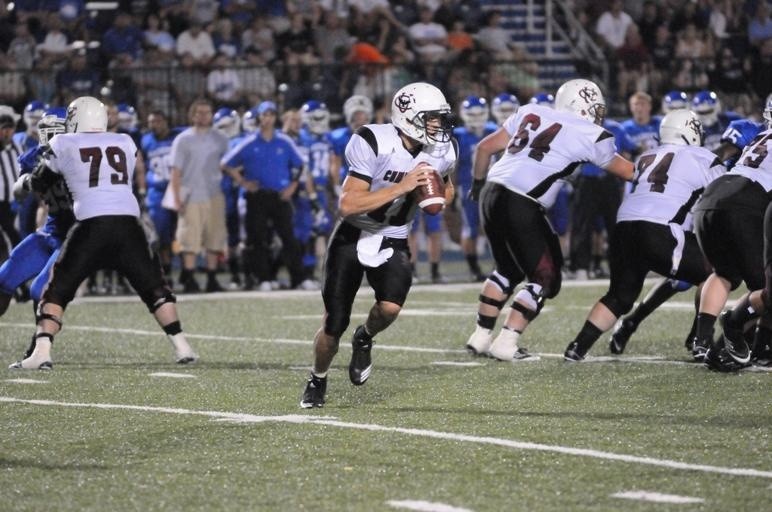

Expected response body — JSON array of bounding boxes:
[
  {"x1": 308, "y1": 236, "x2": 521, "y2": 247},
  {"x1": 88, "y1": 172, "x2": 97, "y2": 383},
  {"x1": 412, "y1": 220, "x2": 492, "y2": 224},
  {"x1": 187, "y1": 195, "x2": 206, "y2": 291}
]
[{"x1": 190, "y1": 109, "x2": 216, "y2": 117}]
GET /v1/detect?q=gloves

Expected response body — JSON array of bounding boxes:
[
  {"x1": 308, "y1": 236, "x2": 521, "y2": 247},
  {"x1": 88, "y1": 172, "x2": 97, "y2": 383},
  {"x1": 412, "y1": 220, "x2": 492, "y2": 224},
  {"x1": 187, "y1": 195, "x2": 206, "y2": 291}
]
[
  {"x1": 468, "y1": 179, "x2": 486, "y2": 200},
  {"x1": 308, "y1": 194, "x2": 322, "y2": 214}
]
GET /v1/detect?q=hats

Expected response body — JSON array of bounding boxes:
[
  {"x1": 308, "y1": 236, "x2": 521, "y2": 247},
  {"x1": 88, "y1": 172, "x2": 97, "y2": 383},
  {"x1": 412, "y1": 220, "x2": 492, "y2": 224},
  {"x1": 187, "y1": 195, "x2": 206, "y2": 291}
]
[
  {"x1": 0, "y1": 105, "x2": 21, "y2": 120},
  {"x1": 258, "y1": 101, "x2": 279, "y2": 116}
]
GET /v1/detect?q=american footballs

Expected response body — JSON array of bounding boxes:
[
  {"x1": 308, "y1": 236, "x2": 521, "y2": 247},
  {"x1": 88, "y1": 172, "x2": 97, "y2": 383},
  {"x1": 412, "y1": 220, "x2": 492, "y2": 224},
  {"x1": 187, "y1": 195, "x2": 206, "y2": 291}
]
[{"x1": 411, "y1": 162, "x2": 445, "y2": 215}]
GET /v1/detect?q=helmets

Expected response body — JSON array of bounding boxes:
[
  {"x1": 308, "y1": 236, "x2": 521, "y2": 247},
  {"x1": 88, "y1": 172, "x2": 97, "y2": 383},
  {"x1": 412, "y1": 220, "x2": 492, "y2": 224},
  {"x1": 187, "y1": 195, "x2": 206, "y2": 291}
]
[
  {"x1": 112, "y1": 103, "x2": 140, "y2": 136},
  {"x1": 552, "y1": 78, "x2": 608, "y2": 129},
  {"x1": 690, "y1": 90, "x2": 722, "y2": 128},
  {"x1": 718, "y1": 116, "x2": 759, "y2": 151},
  {"x1": 341, "y1": 94, "x2": 376, "y2": 131},
  {"x1": 389, "y1": 80, "x2": 458, "y2": 154},
  {"x1": 242, "y1": 107, "x2": 260, "y2": 129},
  {"x1": 22, "y1": 99, "x2": 48, "y2": 134},
  {"x1": 490, "y1": 91, "x2": 521, "y2": 122},
  {"x1": 36, "y1": 106, "x2": 71, "y2": 148},
  {"x1": 299, "y1": 97, "x2": 332, "y2": 137},
  {"x1": 661, "y1": 89, "x2": 690, "y2": 118},
  {"x1": 760, "y1": 96, "x2": 772, "y2": 140},
  {"x1": 658, "y1": 109, "x2": 706, "y2": 148},
  {"x1": 211, "y1": 106, "x2": 242, "y2": 139},
  {"x1": 527, "y1": 90, "x2": 555, "y2": 107},
  {"x1": 459, "y1": 94, "x2": 490, "y2": 129},
  {"x1": 65, "y1": 96, "x2": 109, "y2": 135}
]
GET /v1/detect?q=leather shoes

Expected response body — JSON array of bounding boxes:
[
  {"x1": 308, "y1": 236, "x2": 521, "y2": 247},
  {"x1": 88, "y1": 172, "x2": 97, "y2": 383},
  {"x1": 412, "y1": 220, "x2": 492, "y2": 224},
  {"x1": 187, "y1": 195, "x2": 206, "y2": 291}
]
[
  {"x1": 204, "y1": 281, "x2": 228, "y2": 293},
  {"x1": 182, "y1": 284, "x2": 201, "y2": 294}
]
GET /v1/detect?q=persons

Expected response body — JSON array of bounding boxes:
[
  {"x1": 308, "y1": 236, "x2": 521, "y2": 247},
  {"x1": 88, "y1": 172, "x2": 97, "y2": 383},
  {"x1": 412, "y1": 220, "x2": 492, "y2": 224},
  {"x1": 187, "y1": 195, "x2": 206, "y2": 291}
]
[
  {"x1": 330, "y1": 94, "x2": 373, "y2": 196},
  {"x1": 100, "y1": 11, "x2": 136, "y2": 75},
  {"x1": 566, "y1": 109, "x2": 727, "y2": 362},
  {"x1": 716, "y1": 41, "x2": 745, "y2": 89},
  {"x1": 468, "y1": 79, "x2": 639, "y2": 360},
  {"x1": 218, "y1": 103, "x2": 308, "y2": 292},
  {"x1": 353, "y1": 0, "x2": 409, "y2": 45},
  {"x1": 257, "y1": 0, "x2": 291, "y2": 29},
  {"x1": 644, "y1": 0, "x2": 706, "y2": 32},
  {"x1": 445, "y1": 20, "x2": 474, "y2": 54},
  {"x1": 490, "y1": 44, "x2": 546, "y2": 100},
  {"x1": 0, "y1": 108, "x2": 73, "y2": 360},
  {"x1": 279, "y1": 47, "x2": 314, "y2": 101},
  {"x1": 670, "y1": 57, "x2": 710, "y2": 90},
  {"x1": 490, "y1": 95, "x2": 518, "y2": 126},
  {"x1": 42, "y1": 20, "x2": 67, "y2": 60},
  {"x1": 455, "y1": 98, "x2": 507, "y2": 281},
  {"x1": 677, "y1": 23, "x2": 706, "y2": 62},
  {"x1": 300, "y1": 83, "x2": 456, "y2": 409},
  {"x1": 596, "y1": 1, "x2": 641, "y2": 89},
  {"x1": 10, "y1": 97, "x2": 201, "y2": 368},
  {"x1": 214, "y1": 0, "x2": 260, "y2": 29},
  {"x1": 108, "y1": 52, "x2": 138, "y2": 106},
  {"x1": 2, "y1": 51, "x2": 26, "y2": 105},
  {"x1": 173, "y1": 52, "x2": 205, "y2": 108},
  {"x1": 52, "y1": 0, "x2": 80, "y2": 44},
  {"x1": 170, "y1": 101, "x2": 229, "y2": 293},
  {"x1": 694, "y1": 94, "x2": 747, "y2": 148},
  {"x1": 706, "y1": 2, "x2": 731, "y2": 40},
  {"x1": 135, "y1": 110, "x2": 192, "y2": 292},
  {"x1": 208, "y1": 53, "x2": 240, "y2": 105},
  {"x1": 285, "y1": 0, "x2": 351, "y2": 35},
  {"x1": 308, "y1": 9, "x2": 356, "y2": 63},
  {"x1": 0, "y1": 104, "x2": 34, "y2": 303},
  {"x1": 183, "y1": 0, "x2": 220, "y2": 29},
  {"x1": 60, "y1": 52, "x2": 94, "y2": 104},
  {"x1": 331, "y1": 45, "x2": 366, "y2": 103},
  {"x1": 176, "y1": 18, "x2": 214, "y2": 72},
  {"x1": 29, "y1": 56, "x2": 59, "y2": 109},
  {"x1": 239, "y1": 19, "x2": 274, "y2": 64},
  {"x1": 409, "y1": 7, "x2": 449, "y2": 74},
  {"x1": 380, "y1": 28, "x2": 416, "y2": 81},
  {"x1": 6, "y1": 22, "x2": 37, "y2": 65},
  {"x1": 142, "y1": 10, "x2": 173, "y2": 55},
  {"x1": 644, "y1": 62, "x2": 673, "y2": 111},
  {"x1": 747, "y1": 2, "x2": 770, "y2": 44},
  {"x1": 649, "y1": 24, "x2": 679, "y2": 77},
  {"x1": 290, "y1": 104, "x2": 344, "y2": 289},
  {"x1": 276, "y1": 14, "x2": 320, "y2": 79},
  {"x1": 691, "y1": 93, "x2": 772, "y2": 364},
  {"x1": 751, "y1": 37, "x2": 771, "y2": 87},
  {"x1": 662, "y1": 92, "x2": 688, "y2": 114},
  {"x1": 444, "y1": 58, "x2": 476, "y2": 101},
  {"x1": 208, "y1": 18, "x2": 245, "y2": 60},
  {"x1": 478, "y1": 10, "x2": 522, "y2": 65},
  {"x1": 622, "y1": 91, "x2": 664, "y2": 154}
]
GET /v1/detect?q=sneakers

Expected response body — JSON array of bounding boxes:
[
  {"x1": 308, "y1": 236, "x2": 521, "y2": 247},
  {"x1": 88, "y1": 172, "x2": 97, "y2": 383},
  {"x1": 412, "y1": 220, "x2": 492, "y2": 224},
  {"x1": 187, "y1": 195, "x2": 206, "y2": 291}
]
[
  {"x1": 115, "y1": 285, "x2": 133, "y2": 298},
  {"x1": 102, "y1": 285, "x2": 113, "y2": 297},
  {"x1": 348, "y1": 326, "x2": 376, "y2": 387},
  {"x1": 7, "y1": 348, "x2": 54, "y2": 372},
  {"x1": 300, "y1": 280, "x2": 313, "y2": 291},
  {"x1": 409, "y1": 272, "x2": 419, "y2": 286},
  {"x1": 229, "y1": 279, "x2": 239, "y2": 292},
  {"x1": 259, "y1": 280, "x2": 271, "y2": 294},
  {"x1": 609, "y1": 324, "x2": 631, "y2": 355},
  {"x1": 470, "y1": 272, "x2": 490, "y2": 286},
  {"x1": 431, "y1": 274, "x2": 452, "y2": 284},
  {"x1": 717, "y1": 308, "x2": 751, "y2": 366},
  {"x1": 298, "y1": 370, "x2": 328, "y2": 412},
  {"x1": 165, "y1": 336, "x2": 200, "y2": 365},
  {"x1": 703, "y1": 348, "x2": 720, "y2": 372},
  {"x1": 716, "y1": 353, "x2": 746, "y2": 375},
  {"x1": 83, "y1": 284, "x2": 97, "y2": 296},
  {"x1": 270, "y1": 281, "x2": 279, "y2": 290},
  {"x1": 465, "y1": 329, "x2": 494, "y2": 358},
  {"x1": 684, "y1": 331, "x2": 701, "y2": 351},
  {"x1": 563, "y1": 339, "x2": 586, "y2": 363},
  {"x1": 748, "y1": 354, "x2": 771, "y2": 373},
  {"x1": 488, "y1": 337, "x2": 542, "y2": 364},
  {"x1": 689, "y1": 335, "x2": 714, "y2": 365},
  {"x1": 587, "y1": 266, "x2": 612, "y2": 281}
]
